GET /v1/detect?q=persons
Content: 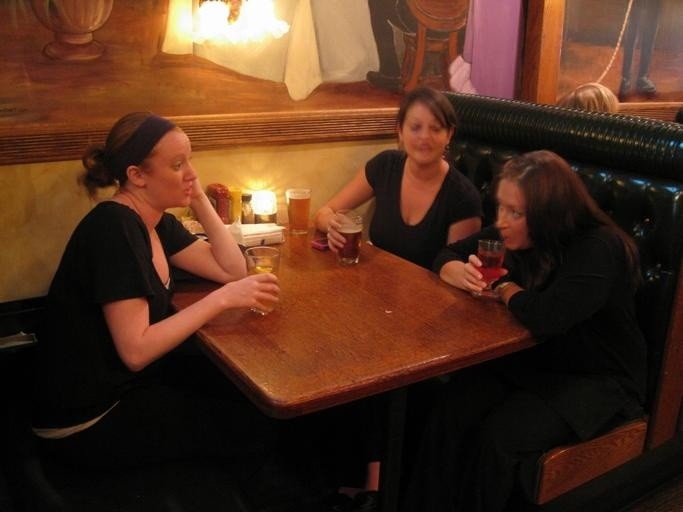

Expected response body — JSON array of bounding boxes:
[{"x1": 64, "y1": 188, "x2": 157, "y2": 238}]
[
  {"x1": 619, "y1": 0, "x2": 661, "y2": 97},
  {"x1": 556, "y1": 82, "x2": 619, "y2": 114},
  {"x1": 33, "y1": 114, "x2": 281, "y2": 512},
  {"x1": 366, "y1": 0, "x2": 466, "y2": 95},
  {"x1": 313, "y1": 87, "x2": 484, "y2": 270},
  {"x1": 399, "y1": 147, "x2": 648, "y2": 512}
]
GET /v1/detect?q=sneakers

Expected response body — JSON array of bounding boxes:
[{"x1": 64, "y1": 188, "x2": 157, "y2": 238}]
[
  {"x1": 637, "y1": 79, "x2": 656, "y2": 93},
  {"x1": 367, "y1": 72, "x2": 401, "y2": 91},
  {"x1": 620, "y1": 81, "x2": 631, "y2": 97}
]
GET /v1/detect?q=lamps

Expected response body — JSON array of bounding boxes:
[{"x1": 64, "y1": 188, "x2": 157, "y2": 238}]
[{"x1": 250, "y1": 189, "x2": 278, "y2": 223}]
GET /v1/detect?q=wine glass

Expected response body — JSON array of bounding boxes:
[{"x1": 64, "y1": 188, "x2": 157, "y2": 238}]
[{"x1": 470, "y1": 239, "x2": 509, "y2": 299}]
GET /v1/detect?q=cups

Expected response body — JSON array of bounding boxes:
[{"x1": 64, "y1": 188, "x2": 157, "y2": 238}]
[
  {"x1": 284, "y1": 188, "x2": 312, "y2": 234},
  {"x1": 333, "y1": 208, "x2": 364, "y2": 266},
  {"x1": 243, "y1": 246, "x2": 282, "y2": 316}
]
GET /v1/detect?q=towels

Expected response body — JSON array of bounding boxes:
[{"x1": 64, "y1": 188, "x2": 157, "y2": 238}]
[{"x1": 223, "y1": 221, "x2": 286, "y2": 247}]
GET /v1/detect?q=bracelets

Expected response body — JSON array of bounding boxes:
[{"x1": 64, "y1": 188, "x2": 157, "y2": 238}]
[{"x1": 494, "y1": 281, "x2": 514, "y2": 293}]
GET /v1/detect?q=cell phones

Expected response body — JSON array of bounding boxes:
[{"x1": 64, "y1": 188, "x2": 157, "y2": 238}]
[{"x1": 311, "y1": 238, "x2": 329, "y2": 251}]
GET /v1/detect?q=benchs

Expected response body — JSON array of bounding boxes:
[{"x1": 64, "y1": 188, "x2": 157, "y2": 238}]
[
  {"x1": 442, "y1": 92, "x2": 683, "y2": 186},
  {"x1": 442, "y1": 144, "x2": 683, "y2": 512},
  {"x1": 0, "y1": 332, "x2": 254, "y2": 511}
]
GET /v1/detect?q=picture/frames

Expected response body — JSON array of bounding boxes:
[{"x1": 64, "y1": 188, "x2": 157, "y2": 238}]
[
  {"x1": 525, "y1": 0, "x2": 683, "y2": 126},
  {"x1": 0, "y1": 0, "x2": 541, "y2": 169}
]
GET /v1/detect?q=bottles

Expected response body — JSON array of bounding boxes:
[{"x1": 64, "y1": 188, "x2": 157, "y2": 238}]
[{"x1": 230, "y1": 190, "x2": 253, "y2": 224}]
